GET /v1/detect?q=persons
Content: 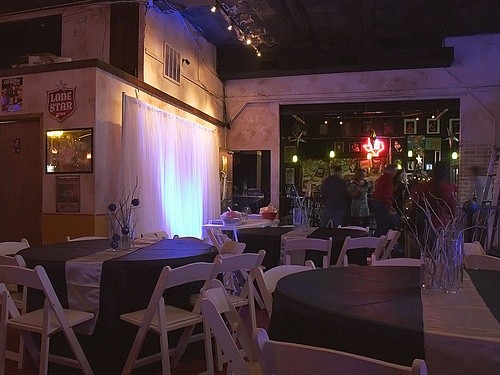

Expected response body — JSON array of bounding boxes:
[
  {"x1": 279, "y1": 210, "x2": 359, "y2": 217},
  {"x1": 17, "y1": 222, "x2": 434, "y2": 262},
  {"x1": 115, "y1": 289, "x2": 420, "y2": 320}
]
[{"x1": 318, "y1": 164, "x2": 459, "y2": 265}]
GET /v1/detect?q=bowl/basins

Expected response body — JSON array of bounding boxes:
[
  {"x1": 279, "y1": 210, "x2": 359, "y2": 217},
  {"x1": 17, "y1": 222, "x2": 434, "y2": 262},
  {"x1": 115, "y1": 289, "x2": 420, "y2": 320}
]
[{"x1": 261, "y1": 213, "x2": 277, "y2": 221}]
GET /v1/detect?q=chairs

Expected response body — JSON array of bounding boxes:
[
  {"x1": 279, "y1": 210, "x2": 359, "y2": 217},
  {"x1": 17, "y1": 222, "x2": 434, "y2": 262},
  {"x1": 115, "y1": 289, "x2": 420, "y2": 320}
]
[{"x1": 0, "y1": 220, "x2": 500, "y2": 375}]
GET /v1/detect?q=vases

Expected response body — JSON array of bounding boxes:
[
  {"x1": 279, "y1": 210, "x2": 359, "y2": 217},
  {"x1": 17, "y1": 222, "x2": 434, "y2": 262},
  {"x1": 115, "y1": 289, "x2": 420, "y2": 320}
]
[
  {"x1": 293, "y1": 209, "x2": 310, "y2": 228},
  {"x1": 420, "y1": 230, "x2": 465, "y2": 293},
  {"x1": 109, "y1": 212, "x2": 134, "y2": 252}
]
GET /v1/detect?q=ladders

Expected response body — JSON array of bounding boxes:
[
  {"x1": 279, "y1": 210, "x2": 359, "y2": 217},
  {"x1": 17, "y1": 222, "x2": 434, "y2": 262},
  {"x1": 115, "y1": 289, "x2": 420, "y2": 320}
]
[{"x1": 471, "y1": 145, "x2": 500, "y2": 255}]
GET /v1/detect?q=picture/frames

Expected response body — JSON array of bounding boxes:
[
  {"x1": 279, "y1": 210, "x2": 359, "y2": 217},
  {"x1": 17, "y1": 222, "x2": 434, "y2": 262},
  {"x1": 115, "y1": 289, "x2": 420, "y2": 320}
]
[
  {"x1": 348, "y1": 141, "x2": 362, "y2": 153},
  {"x1": 404, "y1": 119, "x2": 418, "y2": 135},
  {"x1": 449, "y1": 118, "x2": 460, "y2": 135},
  {"x1": 45, "y1": 127, "x2": 94, "y2": 174},
  {"x1": 427, "y1": 118, "x2": 440, "y2": 135}
]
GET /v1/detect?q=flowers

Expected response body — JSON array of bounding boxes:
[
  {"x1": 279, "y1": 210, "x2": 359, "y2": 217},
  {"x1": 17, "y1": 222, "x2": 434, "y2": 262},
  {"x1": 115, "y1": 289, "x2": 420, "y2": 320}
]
[
  {"x1": 290, "y1": 181, "x2": 313, "y2": 230},
  {"x1": 105, "y1": 175, "x2": 143, "y2": 248},
  {"x1": 388, "y1": 167, "x2": 496, "y2": 291}
]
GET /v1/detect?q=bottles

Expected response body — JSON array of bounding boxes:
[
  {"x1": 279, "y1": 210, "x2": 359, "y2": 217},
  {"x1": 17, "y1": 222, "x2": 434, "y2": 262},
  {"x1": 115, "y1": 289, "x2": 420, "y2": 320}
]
[{"x1": 242, "y1": 183, "x2": 247, "y2": 196}]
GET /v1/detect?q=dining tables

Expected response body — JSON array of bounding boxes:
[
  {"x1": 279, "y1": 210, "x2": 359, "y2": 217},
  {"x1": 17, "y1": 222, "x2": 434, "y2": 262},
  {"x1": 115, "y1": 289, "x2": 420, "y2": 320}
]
[
  {"x1": 275, "y1": 265, "x2": 499, "y2": 375},
  {"x1": 236, "y1": 228, "x2": 368, "y2": 276},
  {"x1": 204, "y1": 219, "x2": 273, "y2": 257},
  {"x1": 16, "y1": 238, "x2": 218, "y2": 367}
]
